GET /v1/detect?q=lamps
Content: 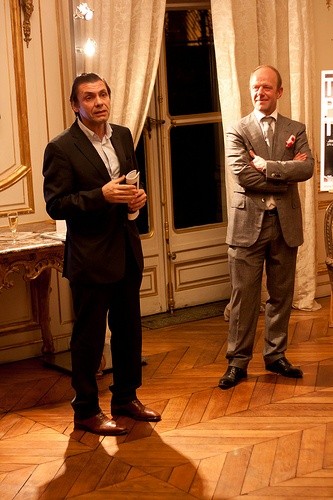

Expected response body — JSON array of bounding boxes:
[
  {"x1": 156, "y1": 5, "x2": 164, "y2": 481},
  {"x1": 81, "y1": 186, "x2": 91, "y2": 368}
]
[{"x1": 70, "y1": 2, "x2": 100, "y2": 59}]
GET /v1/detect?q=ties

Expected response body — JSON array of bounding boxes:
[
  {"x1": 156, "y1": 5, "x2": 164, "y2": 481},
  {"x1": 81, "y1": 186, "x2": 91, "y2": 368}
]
[{"x1": 261, "y1": 117, "x2": 274, "y2": 159}]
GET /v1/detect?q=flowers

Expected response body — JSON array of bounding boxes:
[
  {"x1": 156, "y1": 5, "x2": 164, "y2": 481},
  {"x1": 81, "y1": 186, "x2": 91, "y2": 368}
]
[{"x1": 286, "y1": 134, "x2": 297, "y2": 150}]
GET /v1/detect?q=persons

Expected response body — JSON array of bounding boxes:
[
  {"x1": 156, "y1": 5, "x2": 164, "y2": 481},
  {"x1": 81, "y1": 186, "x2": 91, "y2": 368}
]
[
  {"x1": 42, "y1": 73, "x2": 161, "y2": 436},
  {"x1": 218, "y1": 65, "x2": 314, "y2": 387}
]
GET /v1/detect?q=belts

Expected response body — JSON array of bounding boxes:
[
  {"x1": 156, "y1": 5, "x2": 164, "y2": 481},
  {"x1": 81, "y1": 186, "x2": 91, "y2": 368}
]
[{"x1": 264, "y1": 207, "x2": 279, "y2": 217}]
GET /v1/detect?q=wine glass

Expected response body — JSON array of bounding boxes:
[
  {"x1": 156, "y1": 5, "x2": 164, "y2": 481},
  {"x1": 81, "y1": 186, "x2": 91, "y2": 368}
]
[{"x1": 8, "y1": 211, "x2": 19, "y2": 244}]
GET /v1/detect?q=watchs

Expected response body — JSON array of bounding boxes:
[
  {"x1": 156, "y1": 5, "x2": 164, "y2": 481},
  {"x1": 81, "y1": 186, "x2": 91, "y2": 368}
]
[{"x1": 260, "y1": 167, "x2": 266, "y2": 175}]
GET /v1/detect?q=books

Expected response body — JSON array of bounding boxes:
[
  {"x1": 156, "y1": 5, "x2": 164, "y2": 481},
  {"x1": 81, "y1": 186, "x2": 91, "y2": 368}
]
[
  {"x1": 40, "y1": 230, "x2": 66, "y2": 241},
  {"x1": 125, "y1": 170, "x2": 139, "y2": 221}
]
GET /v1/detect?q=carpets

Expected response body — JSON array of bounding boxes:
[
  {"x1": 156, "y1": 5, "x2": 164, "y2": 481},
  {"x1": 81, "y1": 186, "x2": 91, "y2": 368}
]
[{"x1": 141, "y1": 300, "x2": 227, "y2": 331}]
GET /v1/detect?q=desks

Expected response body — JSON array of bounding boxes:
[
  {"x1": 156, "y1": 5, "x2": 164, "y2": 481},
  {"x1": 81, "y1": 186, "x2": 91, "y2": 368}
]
[{"x1": 1, "y1": 220, "x2": 71, "y2": 364}]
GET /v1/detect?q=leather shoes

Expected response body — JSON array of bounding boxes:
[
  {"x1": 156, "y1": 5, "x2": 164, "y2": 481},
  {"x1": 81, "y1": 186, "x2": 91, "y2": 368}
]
[
  {"x1": 111, "y1": 399, "x2": 161, "y2": 421},
  {"x1": 219, "y1": 366, "x2": 248, "y2": 389},
  {"x1": 265, "y1": 357, "x2": 303, "y2": 379},
  {"x1": 74, "y1": 411, "x2": 128, "y2": 436}
]
